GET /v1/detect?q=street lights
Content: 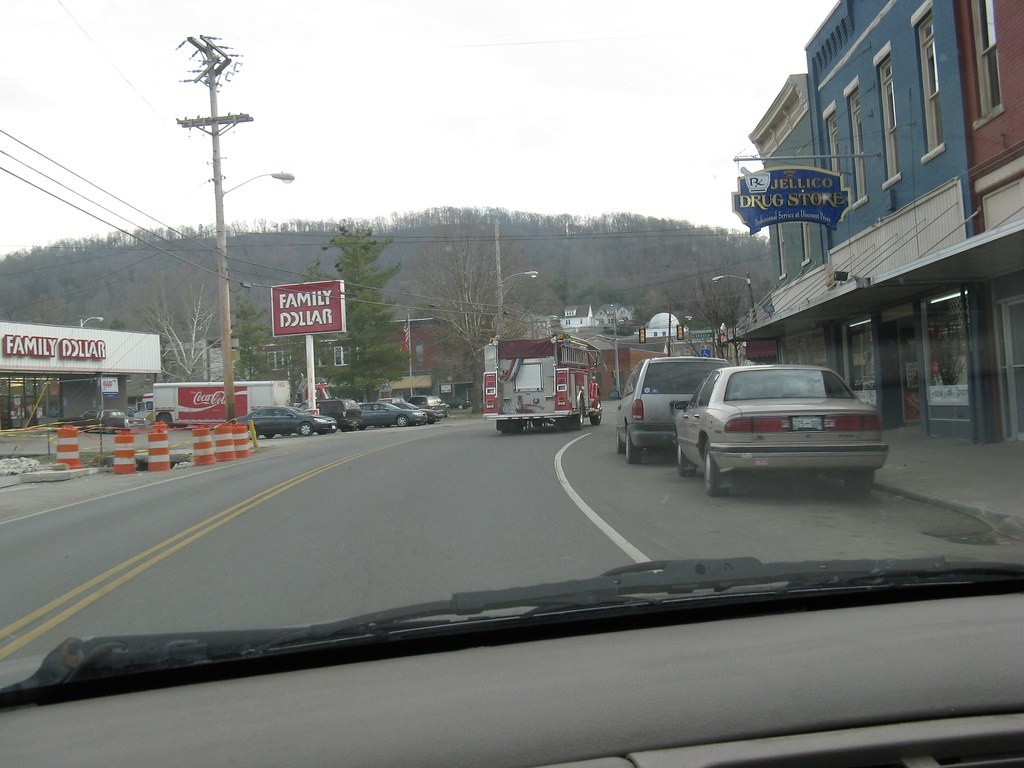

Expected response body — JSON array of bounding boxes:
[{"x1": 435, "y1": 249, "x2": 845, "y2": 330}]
[
  {"x1": 496, "y1": 270, "x2": 539, "y2": 340},
  {"x1": 80, "y1": 316, "x2": 104, "y2": 327},
  {"x1": 712, "y1": 274, "x2": 757, "y2": 324},
  {"x1": 214, "y1": 171, "x2": 295, "y2": 424}
]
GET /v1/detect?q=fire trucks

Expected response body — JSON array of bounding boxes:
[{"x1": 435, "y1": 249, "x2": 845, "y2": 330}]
[{"x1": 481, "y1": 330, "x2": 602, "y2": 435}]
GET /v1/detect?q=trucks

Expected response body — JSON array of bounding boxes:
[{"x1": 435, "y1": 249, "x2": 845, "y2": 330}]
[
  {"x1": 305, "y1": 382, "x2": 332, "y2": 407},
  {"x1": 133, "y1": 380, "x2": 290, "y2": 429}
]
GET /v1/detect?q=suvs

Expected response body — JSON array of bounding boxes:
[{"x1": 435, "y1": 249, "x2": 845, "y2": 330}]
[
  {"x1": 608, "y1": 357, "x2": 730, "y2": 465},
  {"x1": 407, "y1": 396, "x2": 450, "y2": 418},
  {"x1": 298, "y1": 399, "x2": 362, "y2": 432},
  {"x1": 376, "y1": 398, "x2": 406, "y2": 405}
]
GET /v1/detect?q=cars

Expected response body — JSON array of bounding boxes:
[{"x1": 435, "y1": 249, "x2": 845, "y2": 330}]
[
  {"x1": 392, "y1": 401, "x2": 445, "y2": 424},
  {"x1": 444, "y1": 396, "x2": 472, "y2": 409},
  {"x1": 57, "y1": 409, "x2": 146, "y2": 435},
  {"x1": 237, "y1": 406, "x2": 338, "y2": 439},
  {"x1": 357, "y1": 402, "x2": 428, "y2": 430},
  {"x1": 675, "y1": 365, "x2": 889, "y2": 497}
]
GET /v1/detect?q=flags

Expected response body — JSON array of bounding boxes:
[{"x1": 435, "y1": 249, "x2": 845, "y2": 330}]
[{"x1": 399, "y1": 314, "x2": 409, "y2": 351}]
